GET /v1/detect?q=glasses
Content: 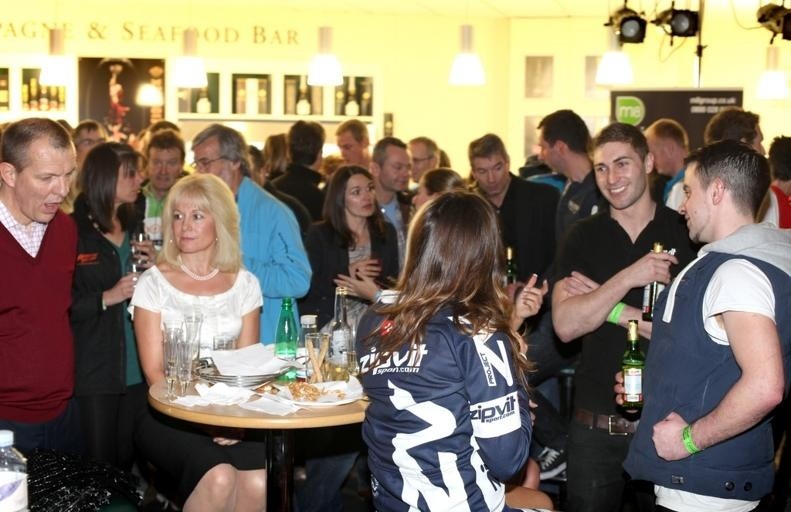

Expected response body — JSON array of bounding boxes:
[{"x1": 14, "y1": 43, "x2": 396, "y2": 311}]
[
  {"x1": 190, "y1": 159, "x2": 212, "y2": 170},
  {"x1": 75, "y1": 137, "x2": 105, "y2": 146}
]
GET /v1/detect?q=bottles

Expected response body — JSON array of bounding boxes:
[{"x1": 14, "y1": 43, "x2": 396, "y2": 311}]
[
  {"x1": 295, "y1": 85, "x2": 311, "y2": 115},
  {"x1": 618, "y1": 318, "x2": 645, "y2": 411},
  {"x1": 343, "y1": 86, "x2": 361, "y2": 116},
  {"x1": 0, "y1": 79, "x2": 7, "y2": 106},
  {"x1": 22, "y1": 76, "x2": 65, "y2": 111},
  {"x1": 273, "y1": 297, "x2": 298, "y2": 382},
  {"x1": 641, "y1": 241, "x2": 669, "y2": 324},
  {"x1": 328, "y1": 284, "x2": 352, "y2": 382},
  {"x1": 294, "y1": 313, "x2": 319, "y2": 378},
  {"x1": 0, "y1": 428, "x2": 29, "y2": 512},
  {"x1": 195, "y1": 86, "x2": 212, "y2": 113},
  {"x1": 502, "y1": 246, "x2": 518, "y2": 285}
]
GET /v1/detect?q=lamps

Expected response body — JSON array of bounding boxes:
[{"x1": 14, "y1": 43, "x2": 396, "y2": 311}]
[
  {"x1": 603, "y1": 0, "x2": 647, "y2": 44},
  {"x1": 594, "y1": 32, "x2": 634, "y2": 85},
  {"x1": 38, "y1": 28, "x2": 77, "y2": 85},
  {"x1": 755, "y1": 46, "x2": 790, "y2": 98},
  {"x1": 649, "y1": 0, "x2": 699, "y2": 46},
  {"x1": 171, "y1": 29, "x2": 208, "y2": 88},
  {"x1": 756, "y1": 0, "x2": 791, "y2": 44},
  {"x1": 306, "y1": 26, "x2": 344, "y2": 88},
  {"x1": 448, "y1": 26, "x2": 486, "y2": 85}
]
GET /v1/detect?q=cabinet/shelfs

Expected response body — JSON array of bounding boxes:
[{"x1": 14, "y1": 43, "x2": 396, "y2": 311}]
[
  {"x1": 179, "y1": 73, "x2": 373, "y2": 159},
  {"x1": 0, "y1": 68, "x2": 66, "y2": 135}
]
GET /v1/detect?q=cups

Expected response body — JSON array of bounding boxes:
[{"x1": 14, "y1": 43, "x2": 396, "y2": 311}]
[
  {"x1": 304, "y1": 332, "x2": 329, "y2": 383},
  {"x1": 125, "y1": 263, "x2": 141, "y2": 275},
  {"x1": 213, "y1": 335, "x2": 238, "y2": 352},
  {"x1": 128, "y1": 231, "x2": 151, "y2": 266}
]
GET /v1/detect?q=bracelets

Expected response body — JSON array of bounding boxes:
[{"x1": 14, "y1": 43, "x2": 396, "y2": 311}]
[{"x1": 682, "y1": 423, "x2": 702, "y2": 454}]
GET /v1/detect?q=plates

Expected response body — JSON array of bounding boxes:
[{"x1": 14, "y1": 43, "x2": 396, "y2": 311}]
[
  {"x1": 275, "y1": 381, "x2": 363, "y2": 409},
  {"x1": 198, "y1": 366, "x2": 275, "y2": 390}
]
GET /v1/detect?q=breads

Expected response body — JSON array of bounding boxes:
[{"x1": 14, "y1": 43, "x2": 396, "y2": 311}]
[{"x1": 290, "y1": 379, "x2": 319, "y2": 401}]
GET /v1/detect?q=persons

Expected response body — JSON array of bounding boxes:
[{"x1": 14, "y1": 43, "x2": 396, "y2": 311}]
[
  {"x1": 1, "y1": 106, "x2": 613, "y2": 512},
  {"x1": 642, "y1": 116, "x2": 692, "y2": 215},
  {"x1": 705, "y1": 108, "x2": 767, "y2": 161},
  {"x1": 756, "y1": 135, "x2": 791, "y2": 228},
  {"x1": 544, "y1": 122, "x2": 709, "y2": 511},
  {"x1": 101, "y1": 64, "x2": 134, "y2": 139},
  {"x1": 613, "y1": 140, "x2": 790, "y2": 512}
]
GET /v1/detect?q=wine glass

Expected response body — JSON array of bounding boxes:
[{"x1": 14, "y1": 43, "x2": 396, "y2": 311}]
[{"x1": 161, "y1": 306, "x2": 204, "y2": 400}]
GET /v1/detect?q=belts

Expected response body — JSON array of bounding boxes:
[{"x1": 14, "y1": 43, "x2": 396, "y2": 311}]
[{"x1": 575, "y1": 407, "x2": 640, "y2": 436}]
